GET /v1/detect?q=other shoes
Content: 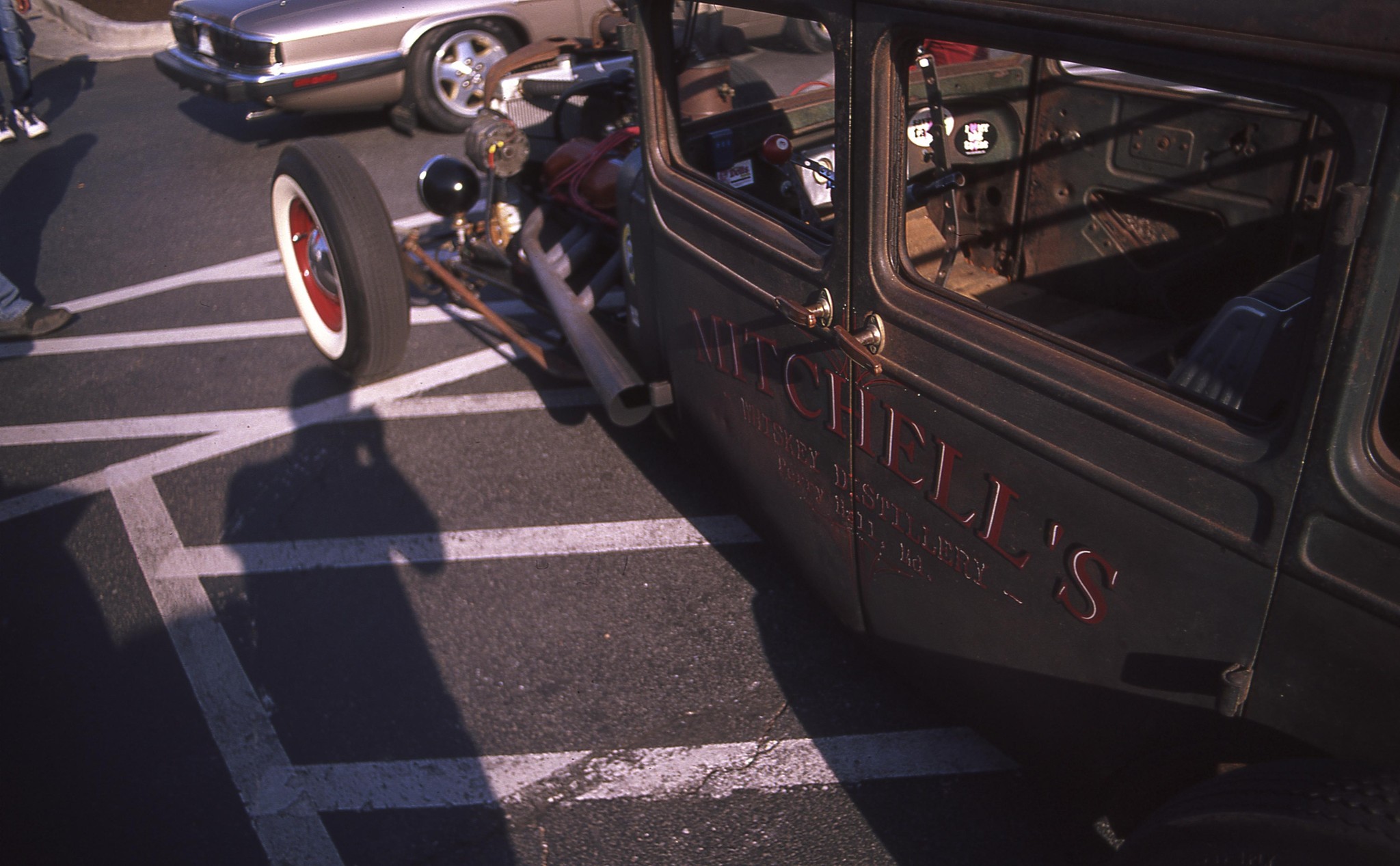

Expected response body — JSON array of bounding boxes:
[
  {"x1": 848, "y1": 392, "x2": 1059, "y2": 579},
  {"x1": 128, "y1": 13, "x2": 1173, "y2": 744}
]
[{"x1": 0, "y1": 306, "x2": 69, "y2": 336}]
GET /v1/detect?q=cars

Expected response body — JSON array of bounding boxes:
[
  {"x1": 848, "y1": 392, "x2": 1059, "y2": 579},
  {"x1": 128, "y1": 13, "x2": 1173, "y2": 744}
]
[{"x1": 150, "y1": 0, "x2": 722, "y2": 136}]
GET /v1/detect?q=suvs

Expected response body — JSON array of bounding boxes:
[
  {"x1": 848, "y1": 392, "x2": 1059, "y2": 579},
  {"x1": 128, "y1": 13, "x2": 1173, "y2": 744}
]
[{"x1": 277, "y1": 0, "x2": 1399, "y2": 866}]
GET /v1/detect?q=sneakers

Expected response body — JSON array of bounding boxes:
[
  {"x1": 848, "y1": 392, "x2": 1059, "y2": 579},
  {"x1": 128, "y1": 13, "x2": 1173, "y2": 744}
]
[
  {"x1": 13, "y1": 108, "x2": 49, "y2": 139},
  {"x1": 0, "y1": 116, "x2": 16, "y2": 142}
]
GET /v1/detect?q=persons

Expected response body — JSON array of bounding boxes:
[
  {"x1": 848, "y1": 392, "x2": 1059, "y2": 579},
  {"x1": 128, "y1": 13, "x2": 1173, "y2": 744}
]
[
  {"x1": 0, "y1": 269, "x2": 72, "y2": 342},
  {"x1": 0, "y1": 0, "x2": 51, "y2": 144}
]
